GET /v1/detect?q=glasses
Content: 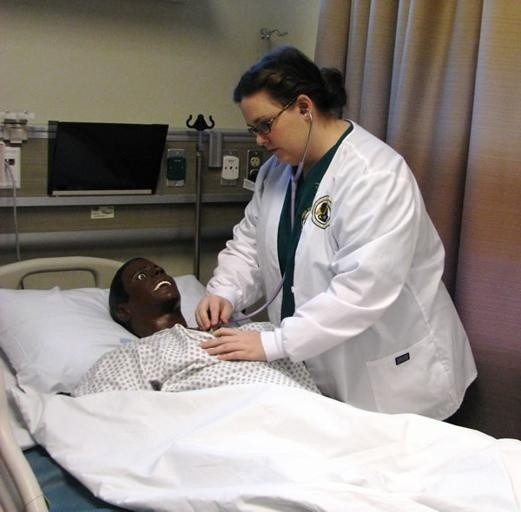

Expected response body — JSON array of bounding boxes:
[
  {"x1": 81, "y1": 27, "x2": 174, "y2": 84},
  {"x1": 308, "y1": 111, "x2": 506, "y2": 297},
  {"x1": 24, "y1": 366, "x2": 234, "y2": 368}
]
[{"x1": 248, "y1": 95, "x2": 299, "y2": 137}]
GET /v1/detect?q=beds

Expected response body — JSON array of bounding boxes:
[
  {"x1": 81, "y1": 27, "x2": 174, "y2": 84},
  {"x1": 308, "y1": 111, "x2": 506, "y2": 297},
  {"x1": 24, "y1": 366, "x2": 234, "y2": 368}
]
[{"x1": 0, "y1": 257, "x2": 521, "y2": 511}]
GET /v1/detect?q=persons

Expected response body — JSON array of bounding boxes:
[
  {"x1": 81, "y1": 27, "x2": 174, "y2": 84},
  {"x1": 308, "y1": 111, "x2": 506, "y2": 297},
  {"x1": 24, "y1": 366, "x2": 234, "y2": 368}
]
[
  {"x1": 69, "y1": 256, "x2": 324, "y2": 398},
  {"x1": 194, "y1": 46, "x2": 479, "y2": 429}
]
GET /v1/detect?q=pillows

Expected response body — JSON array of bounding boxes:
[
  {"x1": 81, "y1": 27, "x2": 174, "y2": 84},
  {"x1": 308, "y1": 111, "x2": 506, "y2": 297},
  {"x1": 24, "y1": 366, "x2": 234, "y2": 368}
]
[{"x1": 0, "y1": 273, "x2": 239, "y2": 396}]
